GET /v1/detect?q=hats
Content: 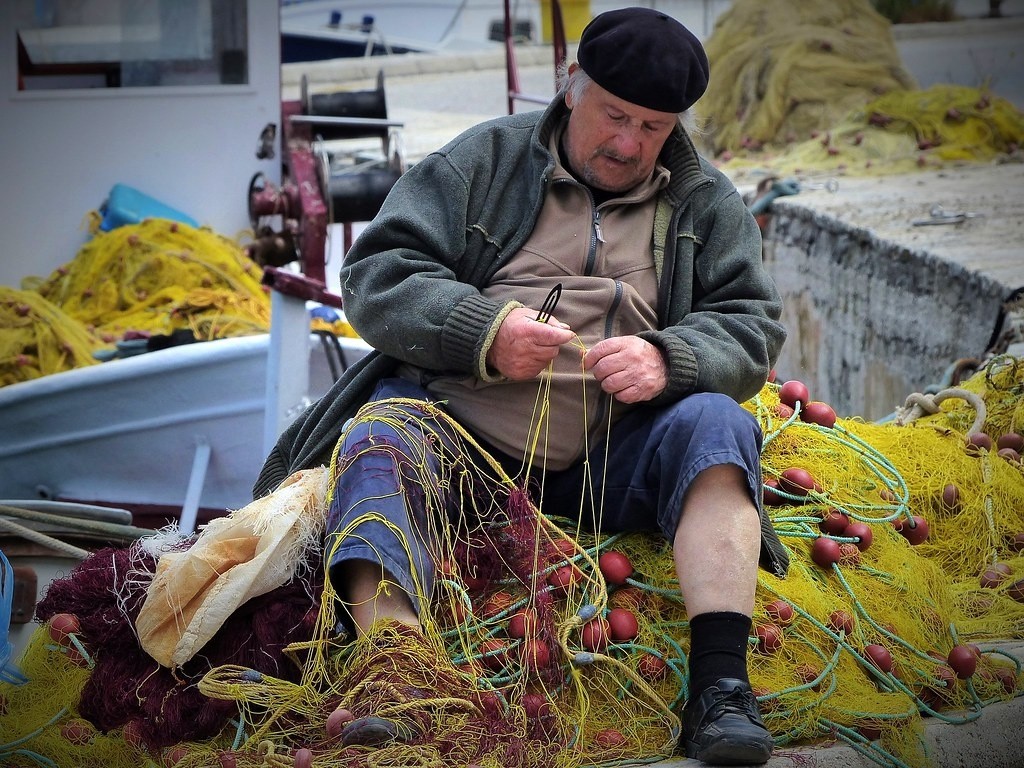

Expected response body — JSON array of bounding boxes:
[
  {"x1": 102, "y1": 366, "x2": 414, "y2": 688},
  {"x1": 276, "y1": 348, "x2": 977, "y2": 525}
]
[{"x1": 577, "y1": 8, "x2": 710, "y2": 114}]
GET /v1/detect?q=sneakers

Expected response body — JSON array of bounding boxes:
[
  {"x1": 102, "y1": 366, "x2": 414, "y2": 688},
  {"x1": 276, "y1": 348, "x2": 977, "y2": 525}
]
[
  {"x1": 341, "y1": 679, "x2": 419, "y2": 743},
  {"x1": 677, "y1": 679, "x2": 772, "y2": 765}
]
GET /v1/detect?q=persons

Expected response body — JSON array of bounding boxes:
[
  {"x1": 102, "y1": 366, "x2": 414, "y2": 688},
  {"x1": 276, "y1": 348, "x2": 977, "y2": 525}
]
[{"x1": 253, "y1": 6, "x2": 790, "y2": 763}]
